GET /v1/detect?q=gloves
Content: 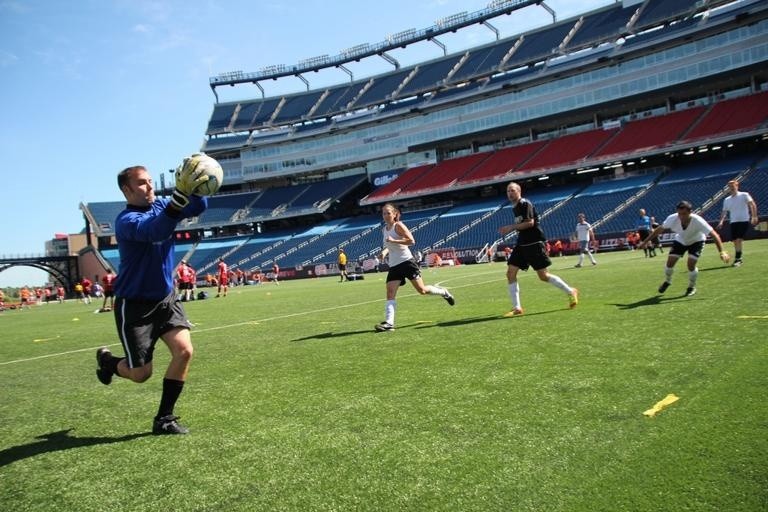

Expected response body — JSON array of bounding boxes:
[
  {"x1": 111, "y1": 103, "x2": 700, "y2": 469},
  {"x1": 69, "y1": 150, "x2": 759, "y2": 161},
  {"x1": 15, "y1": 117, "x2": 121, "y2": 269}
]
[
  {"x1": 172, "y1": 158, "x2": 209, "y2": 207},
  {"x1": 719, "y1": 251, "x2": 728, "y2": 263},
  {"x1": 639, "y1": 242, "x2": 647, "y2": 248}
]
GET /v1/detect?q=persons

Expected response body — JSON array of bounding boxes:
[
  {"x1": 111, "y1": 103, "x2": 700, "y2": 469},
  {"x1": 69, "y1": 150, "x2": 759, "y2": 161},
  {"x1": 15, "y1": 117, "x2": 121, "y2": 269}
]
[
  {"x1": 20, "y1": 288, "x2": 31, "y2": 310},
  {"x1": 501, "y1": 182, "x2": 578, "y2": 317},
  {"x1": 715, "y1": 180, "x2": 758, "y2": 267},
  {"x1": 216, "y1": 258, "x2": 228, "y2": 297},
  {"x1": 96, "y1": 152, "x2": 212, "y2": 435},
  {"x1": 554, "y1": 239, "x2": 562, "y2": 257},
  {"x1": 415, "y1": 249, "x2": 426, "y2": 268},
  {"x1": 336, "y1": 249, "x2": 350, "y2": 283},
  {"x1": 236, "y1": 267, "x2": 244, "y2": 286},
  {"x1": 182, "y1": 263, "x2": 196, "y2": 302},
  {"x1": 213, "y1": 272, "x2": 220, "y2": 287},
  {"x1": 374, "y1": 204, "x2": 455, "y2": 331},
  {"x1": 174, "y1": 259, "x2": 194, "y2": 302},
  {"x1": 93, "y1": 282, "x2": 103, "y2": 300},
  {"x1": 487, "y1": 247, "x2": 492, "y2": 263},
  {"x1": 626, "y1": 231, "x2": 634, "y2": 250},
  {"x1": 272, "y1": 261, "x2": 280, "y2": 286},
  {"x1": 631, "y1": 234, "x2": 638, "y2": 252},
  {"x1": 633, "y1": 208, "x2": 655, "y2": 258},
  {"x1": 57, "y1": 285, "x2": 65, "y2": 305},
  {"x1": 228, "y1": 268, "x2": 235, "y2": 288},
  {"x1": 253, "y1": 272, "x2": 261, "y2": 286},
  {"x1": 635, "y1": 231, "x2": 640, "y2": 245},
  {"x1": 97, "y1": 268, "x2": 118, "y2": 313},
  {"x1": 504, "y1": 245, "x2": 512, "y2": 262},
  {"x1": 44, "y1": 287, "x2": 52, "y2": 304},
  {"x1": 35, "y1": 288, "x2": 40, "y2": 305},
  {"x1": 81, "y1": 276, "x2": 92, "y2": 305},
  {"x1": 373, "y1": 255, "x2": 379, "y2": 274},
  {"x1": 574, "y1": 213, "x2": 597, "y2": 267},
  {"x1": 649, "y1": 217, "x2": 664, "y2": 256},
  {"x1": 639, "y1": 201, "x2": 730, "y2": 296},
  {"x1": 434, "y1": 252, "x2": 441, "y2": 269},
  {"x1": 544, "y1": 240, "x2": 550, "y2": 255},
  {"x1": 75, "y1": 282, "x2": 84, "y2": 301},
  {"x1": 205, "y1": 273, "x2": 212, "y2": 287}
]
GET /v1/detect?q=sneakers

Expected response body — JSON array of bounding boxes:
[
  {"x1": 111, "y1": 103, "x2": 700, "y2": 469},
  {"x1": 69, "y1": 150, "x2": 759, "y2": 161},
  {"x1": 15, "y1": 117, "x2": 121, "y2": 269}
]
[
  {"x1": 686, "y1": 287, "x2": 696, "y2": 296},
  {"x1": 575, "y1": 264, "x2": 583, "y2": 267},
  {"x1": 591, "y1": 262, "x2": 596, "y2": 266},
  {"x1": 568, "y1": 288, "x2": 577, "y2": 308},
  {"x1": 504, "y1": 307, "x2": 523, "y2": 317},
  {"x1": 152, "y1": 416, "x2": 189, "y2": 434},
  {"x1": 661, "y1": 249, "x2": 664, "y2": 252},
  {"x1": 224, "y1": 294, "x2": 227, "y2": 297},
  {"x1": 654, "y1": 253, "x2": 656, "y2": 256},
  {"x1": 733, "y1": 260, "x2": 743, "y2": 267},
  {"x1": 442, "y1": 288, "x2": 454, "y2": 306},
  {"x1": 649, "y1": 255, "x2": 653, "y2": 258},
  {"x1": 645, "y1": 255, "x2": 648, "y2": 257},
  {"x1": 375, "y1": 322, "x2": 395, "y2": 332},
  {"x1": 659, "y1": 281, "x2": 669, "y2": 293},
  {"x1": 96, "y1": 346, "x2": 112, "y2": 385},
  {"x1": 214, "y1": 294, "x2": 220, "y2": 298}
]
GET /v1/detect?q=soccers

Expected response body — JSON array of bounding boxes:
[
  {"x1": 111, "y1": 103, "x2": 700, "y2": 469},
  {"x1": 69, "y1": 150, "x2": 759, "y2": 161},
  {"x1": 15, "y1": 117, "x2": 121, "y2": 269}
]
[{"x1": 181, "y1": 156, "x2": 225, "y2": 196}]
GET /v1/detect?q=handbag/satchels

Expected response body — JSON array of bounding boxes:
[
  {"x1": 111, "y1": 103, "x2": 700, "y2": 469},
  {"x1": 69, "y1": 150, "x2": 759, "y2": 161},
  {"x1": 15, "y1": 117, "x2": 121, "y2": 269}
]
[
  {"x1": 347, "y1": 276, "x2": 355, "y2": 281},
  {"x1": 356, "y1": 275, "x2": 364, "y2": 280},
  {"x1": 198, "y1": 291, "x2": 208, "y2": 300}
]
